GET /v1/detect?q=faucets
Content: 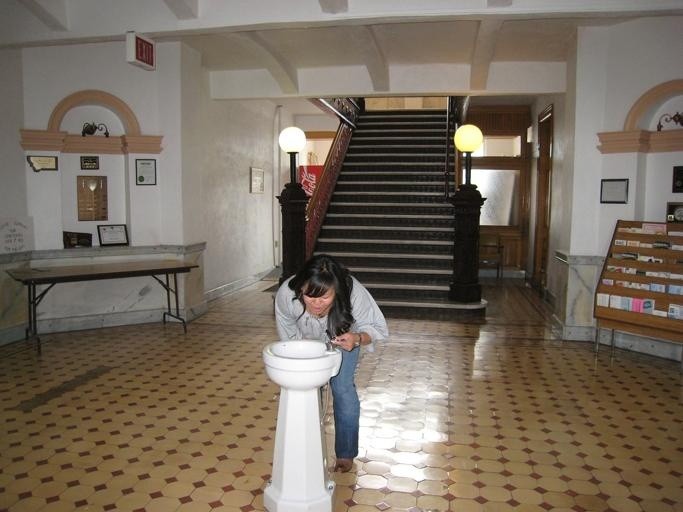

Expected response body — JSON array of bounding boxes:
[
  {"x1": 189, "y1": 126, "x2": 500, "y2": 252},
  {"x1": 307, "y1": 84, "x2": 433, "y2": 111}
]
[{"x1": 324, "y1": 330, "x2": 338, "y2": 352}]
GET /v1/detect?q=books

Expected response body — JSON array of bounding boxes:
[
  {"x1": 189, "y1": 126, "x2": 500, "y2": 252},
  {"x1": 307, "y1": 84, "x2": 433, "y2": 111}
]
[{"x1": 595, "y1": 224, "x2": 683, "y2": 321}]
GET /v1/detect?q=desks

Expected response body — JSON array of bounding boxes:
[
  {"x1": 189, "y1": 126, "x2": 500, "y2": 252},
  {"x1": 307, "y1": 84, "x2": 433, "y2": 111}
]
[{"x1": 6, "y1": 259, "x2": 199, "y2": 355}]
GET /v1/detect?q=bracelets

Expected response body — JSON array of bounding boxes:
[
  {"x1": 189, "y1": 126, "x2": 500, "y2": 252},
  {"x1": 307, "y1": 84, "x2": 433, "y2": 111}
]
[{"x1": 356, "y1": 333, "x2": 361, "y2": 346}]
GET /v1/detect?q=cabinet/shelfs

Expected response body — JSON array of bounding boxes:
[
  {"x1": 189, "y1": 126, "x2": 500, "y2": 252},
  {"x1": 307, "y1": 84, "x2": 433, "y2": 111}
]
[{"x1": 594, "y1": 219, "x2": 683, "y2": 375}]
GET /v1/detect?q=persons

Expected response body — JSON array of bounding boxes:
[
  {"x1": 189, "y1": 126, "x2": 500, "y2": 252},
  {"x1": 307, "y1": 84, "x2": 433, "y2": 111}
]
[{"x1": 274, "y1": 256, "x2": 390, "y2": 472}]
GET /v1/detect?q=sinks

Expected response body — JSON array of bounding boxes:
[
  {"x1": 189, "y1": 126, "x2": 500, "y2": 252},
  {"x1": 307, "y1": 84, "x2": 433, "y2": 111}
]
[{"x1": 262, "y1": 339, "x2": 343, "y2": 391}]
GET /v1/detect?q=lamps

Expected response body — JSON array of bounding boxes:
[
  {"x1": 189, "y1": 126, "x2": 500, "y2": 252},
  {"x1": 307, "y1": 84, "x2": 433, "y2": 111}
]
[
  {"x1": 278, "y1": 126, "x2": 306, "y2": 187},
  {"x1": 453, "y1": 123, "x2": 483, "y2": 190}
]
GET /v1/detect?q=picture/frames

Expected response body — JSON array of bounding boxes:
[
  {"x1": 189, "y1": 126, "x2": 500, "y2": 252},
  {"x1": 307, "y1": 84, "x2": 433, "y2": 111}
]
[
  {"x1": 136, "y1": 159, "x2": 156, "y2": 185},
  {"x1": 98, "y1": 224, "x2": 129, "y2": 246},
  {"x1": 666, "y1": 202, "x2": 683, "y2": 224},
  {"x1": 81, "y1": 156, "x2": 99, "y2": 169},
  {"x1": 251, "y1": 168, "x2": 264, "y2": 193},
  {"x1": 673, "y1": 166, "x2": 683, "y2": 192},
  {"x1": 600, "y1": 179, "x2": 628, "y2": 203}
]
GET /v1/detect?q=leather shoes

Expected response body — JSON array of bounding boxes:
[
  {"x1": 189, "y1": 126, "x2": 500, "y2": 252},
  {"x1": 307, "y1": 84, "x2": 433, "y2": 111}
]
[{"x1": 334, "y1": 457, "x2": 354, "y2": 473}]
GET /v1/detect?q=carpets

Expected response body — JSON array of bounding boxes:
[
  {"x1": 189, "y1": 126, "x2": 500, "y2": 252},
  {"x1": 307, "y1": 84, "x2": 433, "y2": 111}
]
[{"x1": 261, "y1": 266, "x2": 283, "y2": 281}]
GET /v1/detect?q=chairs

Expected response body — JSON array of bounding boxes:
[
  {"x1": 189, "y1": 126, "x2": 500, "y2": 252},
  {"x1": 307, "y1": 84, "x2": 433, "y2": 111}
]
[{"x1": 479, "y1": 232, "x2": 504, "y2": 280}]
